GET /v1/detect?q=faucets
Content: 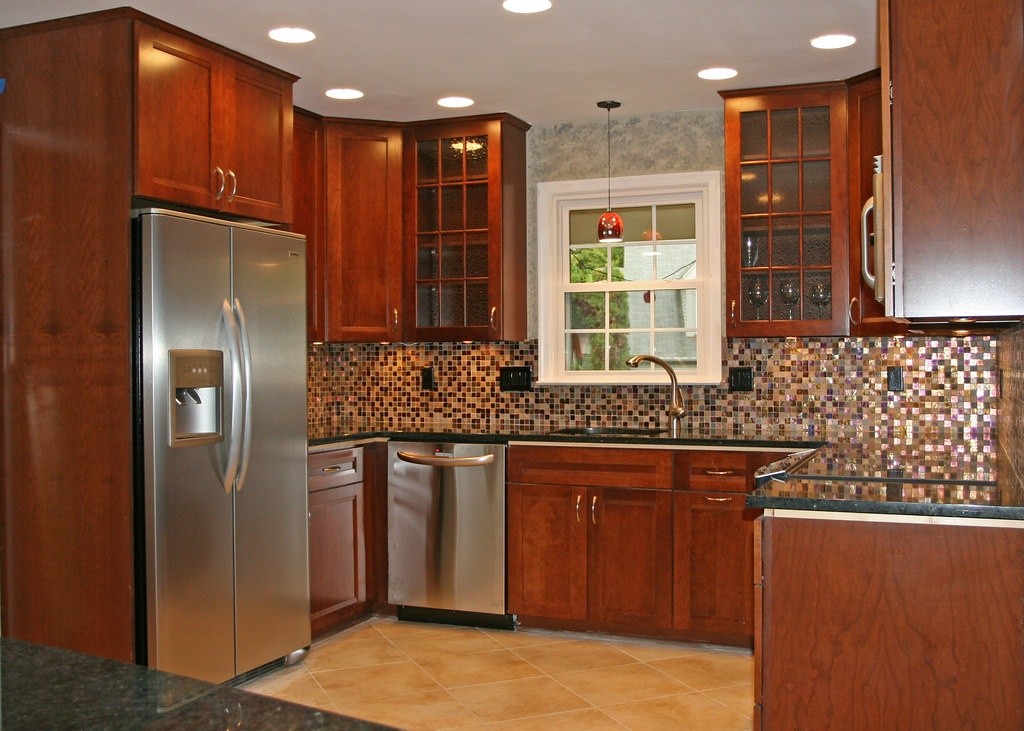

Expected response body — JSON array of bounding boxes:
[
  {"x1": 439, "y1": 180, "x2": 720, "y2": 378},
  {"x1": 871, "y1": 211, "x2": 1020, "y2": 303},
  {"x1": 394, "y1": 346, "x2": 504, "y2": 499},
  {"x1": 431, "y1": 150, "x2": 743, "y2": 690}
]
[{"x1": 627, "y1": 354, "x2": 687, "y2": 439}]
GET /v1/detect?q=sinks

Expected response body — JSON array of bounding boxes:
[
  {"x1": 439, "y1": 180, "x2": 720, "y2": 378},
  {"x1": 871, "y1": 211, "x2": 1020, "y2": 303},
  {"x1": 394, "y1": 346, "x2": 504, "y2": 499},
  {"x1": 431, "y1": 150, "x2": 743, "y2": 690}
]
[{"x1": 543, "y1": 425, "x2": 673, "y2": 440}]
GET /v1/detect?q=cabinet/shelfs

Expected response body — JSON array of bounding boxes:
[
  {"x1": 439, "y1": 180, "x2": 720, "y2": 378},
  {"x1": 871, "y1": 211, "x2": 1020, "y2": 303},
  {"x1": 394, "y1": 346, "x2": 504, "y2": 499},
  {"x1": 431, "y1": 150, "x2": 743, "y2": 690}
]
[
  {"x1": 717, "y1": 0, "x2": 1024, "y2": 339},
  {"x1": 132, "y1": 20, "x2": 528, "y2": 342},
  {"x1": 507, "y1": 446, "x2": 1024, "y2": 731},
  {"x1": 306, "y1": 443, "x2": 373, "y2": 635}
]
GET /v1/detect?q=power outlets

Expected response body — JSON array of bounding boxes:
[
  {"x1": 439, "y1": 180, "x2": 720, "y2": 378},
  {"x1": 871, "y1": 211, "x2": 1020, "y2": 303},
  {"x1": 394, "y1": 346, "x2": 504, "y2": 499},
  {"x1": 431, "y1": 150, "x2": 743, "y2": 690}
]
[
  {"x1": 729, "y1": 367, "x2": 754, "y2": 391},
  {"x1": 500, "y1": 366, "x2": 532, "y2": 391},
  {"x1": 887, "y1": 366, "x2": 903, "y2": 391},
  {"x1": 422, "y1": 368, "x2": 433, "y2": 389}
]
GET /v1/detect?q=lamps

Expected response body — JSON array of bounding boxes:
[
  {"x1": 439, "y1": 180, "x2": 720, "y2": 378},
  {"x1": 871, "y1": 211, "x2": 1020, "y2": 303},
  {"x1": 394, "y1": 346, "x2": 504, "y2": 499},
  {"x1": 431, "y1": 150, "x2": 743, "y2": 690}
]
[{"x1": 597, "y1": 101, "x2": 624, "y2": 242}]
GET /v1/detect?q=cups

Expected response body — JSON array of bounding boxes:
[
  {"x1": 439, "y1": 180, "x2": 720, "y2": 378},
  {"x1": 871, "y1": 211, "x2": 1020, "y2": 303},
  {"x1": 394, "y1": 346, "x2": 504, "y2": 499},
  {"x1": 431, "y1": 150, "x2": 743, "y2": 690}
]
[{"x1": 742, "y1": 236, "x2": 760, "y2": 268}]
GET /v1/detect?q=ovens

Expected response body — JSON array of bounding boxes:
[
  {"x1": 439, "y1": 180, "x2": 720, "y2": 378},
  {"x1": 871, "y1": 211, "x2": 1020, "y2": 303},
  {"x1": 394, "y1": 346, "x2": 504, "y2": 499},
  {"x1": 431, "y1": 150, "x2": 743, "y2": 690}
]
[{"x1": 387, "y1": 432, "x2": 513, "y2": 629}]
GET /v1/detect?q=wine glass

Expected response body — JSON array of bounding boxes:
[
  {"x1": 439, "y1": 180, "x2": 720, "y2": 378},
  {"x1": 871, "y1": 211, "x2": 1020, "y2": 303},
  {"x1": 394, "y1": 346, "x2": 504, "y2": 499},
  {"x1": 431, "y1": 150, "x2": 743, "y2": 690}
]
[
  {"x1": 779, "y1": 280, "x2": 799, "y2": 320},
  {"x1": 810, "y1": 279, "x2": 831, "y2": 320},
  {"x1": 749, "y1": 274, "x2": 769, "y2": 320}
]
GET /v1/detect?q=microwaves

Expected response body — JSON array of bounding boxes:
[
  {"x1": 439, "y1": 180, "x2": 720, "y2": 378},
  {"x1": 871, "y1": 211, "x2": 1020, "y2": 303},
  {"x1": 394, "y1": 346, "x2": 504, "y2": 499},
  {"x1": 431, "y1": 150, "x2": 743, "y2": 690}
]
[{"x1": 862, "y1": 173, "x2": 885, "y2": 305}]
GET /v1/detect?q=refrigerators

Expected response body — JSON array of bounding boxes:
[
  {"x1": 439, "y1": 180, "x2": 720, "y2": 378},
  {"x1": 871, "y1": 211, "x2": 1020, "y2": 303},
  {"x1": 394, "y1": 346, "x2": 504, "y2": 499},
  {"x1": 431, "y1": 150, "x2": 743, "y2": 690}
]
[{"x1": 131, "y1": 209, "x2": 312, "y2": 692}]
